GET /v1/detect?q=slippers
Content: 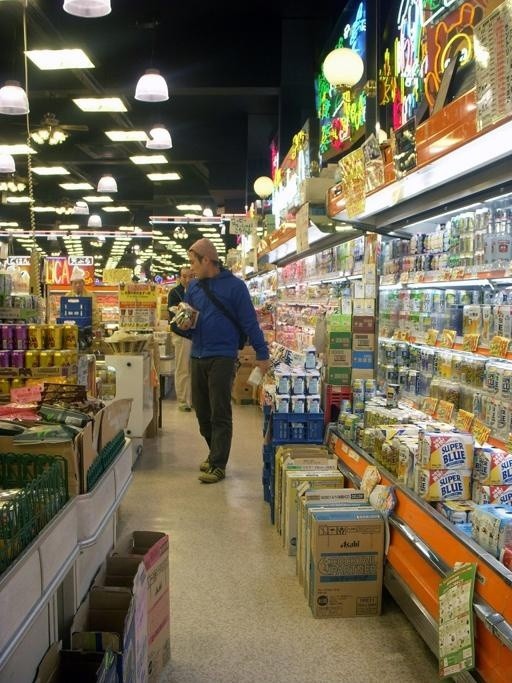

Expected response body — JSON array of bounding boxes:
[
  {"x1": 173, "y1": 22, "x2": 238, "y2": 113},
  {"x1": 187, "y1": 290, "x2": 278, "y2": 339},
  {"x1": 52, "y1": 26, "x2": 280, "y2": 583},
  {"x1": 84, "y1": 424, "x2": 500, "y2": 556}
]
[{"x1": 179, "y1": 405, "x2": 191, "y2": 411}]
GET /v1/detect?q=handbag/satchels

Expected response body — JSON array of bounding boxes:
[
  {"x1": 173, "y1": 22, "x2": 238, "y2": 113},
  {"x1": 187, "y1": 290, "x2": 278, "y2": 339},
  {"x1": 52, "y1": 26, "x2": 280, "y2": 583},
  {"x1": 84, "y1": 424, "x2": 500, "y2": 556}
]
[{"x1": 239, "y1": 332, "x2": 247, "y2": 350}]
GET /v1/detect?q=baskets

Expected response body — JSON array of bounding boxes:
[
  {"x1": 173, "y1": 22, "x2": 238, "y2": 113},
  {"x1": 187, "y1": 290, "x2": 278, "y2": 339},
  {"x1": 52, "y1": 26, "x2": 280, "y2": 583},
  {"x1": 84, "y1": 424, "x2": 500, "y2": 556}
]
[
  {"x1": 56, "y1": 297, "x2": 92, "y2": 325},
  {"x1": 262, "y1": 413, "x2": 324, "y2": 524}
]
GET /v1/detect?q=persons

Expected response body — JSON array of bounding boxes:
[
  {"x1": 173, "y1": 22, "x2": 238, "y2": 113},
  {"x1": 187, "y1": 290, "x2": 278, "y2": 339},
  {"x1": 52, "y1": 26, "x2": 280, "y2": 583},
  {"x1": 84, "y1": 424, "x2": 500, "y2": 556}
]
[
  {"x1": 168, "y1": 265, "x2": 197, "y2": 413},
  {"x1": 174, "y1": 237, "x2": 273, "y2": 484},
  {"x1": 63, "y1": 271, "x2": 97, "y2": 317}
]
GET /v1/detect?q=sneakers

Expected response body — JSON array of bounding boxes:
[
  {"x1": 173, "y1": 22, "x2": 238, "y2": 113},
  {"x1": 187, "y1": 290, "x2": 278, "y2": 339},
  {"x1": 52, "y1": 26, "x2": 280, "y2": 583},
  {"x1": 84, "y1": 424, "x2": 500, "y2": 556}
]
[{"x1": 199, "y1": 458, "x2": 225, "y2": 483}]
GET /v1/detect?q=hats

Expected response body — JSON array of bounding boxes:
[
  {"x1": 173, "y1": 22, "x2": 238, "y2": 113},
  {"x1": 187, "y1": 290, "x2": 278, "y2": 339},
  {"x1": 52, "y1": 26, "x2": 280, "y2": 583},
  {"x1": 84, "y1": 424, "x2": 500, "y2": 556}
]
[{"x1": 189, "y1": 238, "x2": 218, "y2": 261}]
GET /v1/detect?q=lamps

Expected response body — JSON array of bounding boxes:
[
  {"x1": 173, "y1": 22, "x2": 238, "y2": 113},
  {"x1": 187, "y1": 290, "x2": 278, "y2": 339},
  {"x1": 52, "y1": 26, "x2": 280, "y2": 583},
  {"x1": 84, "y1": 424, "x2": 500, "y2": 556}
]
[
  {"x1": 322, "y1": 43, "x2": 368, "y2": 102},
  {"x1": 1, "y1": 0, "x2": 223, "y2": 237}
]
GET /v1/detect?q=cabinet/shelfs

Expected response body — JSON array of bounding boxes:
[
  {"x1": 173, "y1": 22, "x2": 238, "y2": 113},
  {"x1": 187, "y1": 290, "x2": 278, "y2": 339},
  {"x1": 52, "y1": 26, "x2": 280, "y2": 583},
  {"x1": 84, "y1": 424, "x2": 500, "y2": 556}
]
[
  {"x1": 231, "y1": 115, "x2": 512, "y2": 683},
  {"x1": 0, "y1": 354, "x2": 175, "y2": 683}
]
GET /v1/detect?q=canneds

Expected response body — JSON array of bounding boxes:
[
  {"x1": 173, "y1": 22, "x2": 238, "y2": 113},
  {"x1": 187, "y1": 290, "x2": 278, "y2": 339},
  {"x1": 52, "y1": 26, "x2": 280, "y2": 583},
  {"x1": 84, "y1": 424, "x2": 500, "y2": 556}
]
[
  {"x1": 0, "y1": 273, "x2": 116, "y2": 396},
  {"x1": 337, "y1": 208, "x2": 512, "y2": 524}
]
